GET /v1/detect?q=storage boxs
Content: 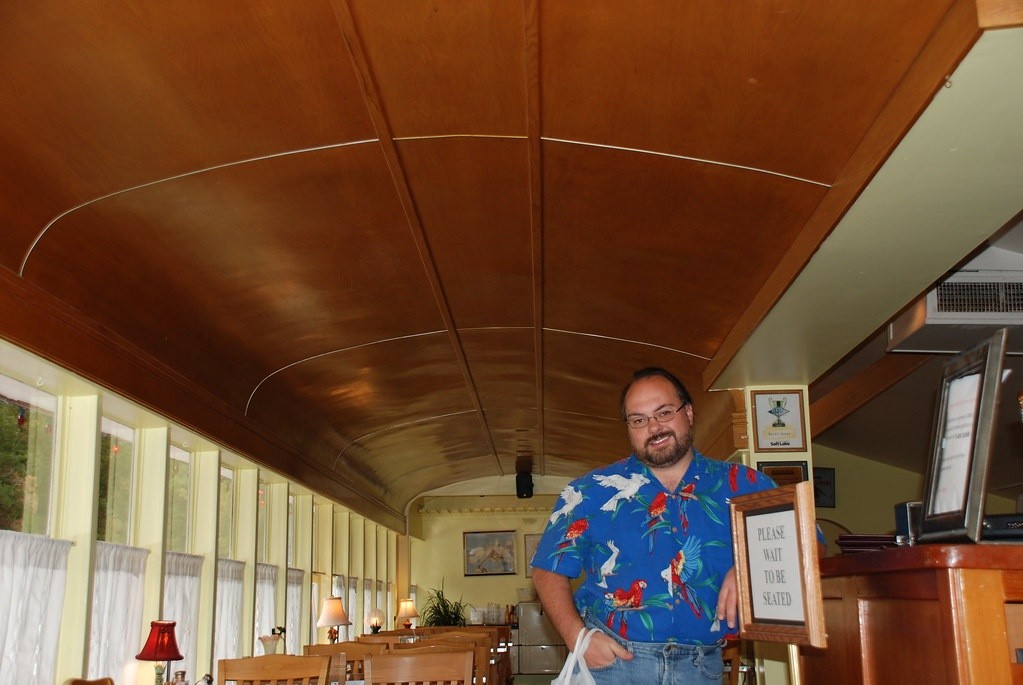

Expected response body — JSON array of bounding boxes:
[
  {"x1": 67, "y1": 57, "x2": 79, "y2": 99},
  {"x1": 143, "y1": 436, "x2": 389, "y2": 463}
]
[{"x1": 511, "y1": 602, "x2": 566, "y2": 675}]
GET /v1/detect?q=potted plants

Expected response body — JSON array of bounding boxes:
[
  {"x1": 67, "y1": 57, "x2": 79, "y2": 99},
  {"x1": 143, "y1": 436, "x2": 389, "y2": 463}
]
[{"x1": 258, "y1": 627, "x2": 285, "y2": 655}]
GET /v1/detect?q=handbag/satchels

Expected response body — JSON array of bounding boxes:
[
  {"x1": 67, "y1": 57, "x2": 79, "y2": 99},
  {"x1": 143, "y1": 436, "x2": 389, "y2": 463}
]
[{"x1": 551, "y1": 626, "x2": 604, "y2": 685}]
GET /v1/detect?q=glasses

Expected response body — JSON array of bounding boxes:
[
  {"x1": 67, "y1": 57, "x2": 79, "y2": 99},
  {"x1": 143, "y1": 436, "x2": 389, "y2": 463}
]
[{"x1": 626, "y1": 401, "x2": 686, "y2": 428}]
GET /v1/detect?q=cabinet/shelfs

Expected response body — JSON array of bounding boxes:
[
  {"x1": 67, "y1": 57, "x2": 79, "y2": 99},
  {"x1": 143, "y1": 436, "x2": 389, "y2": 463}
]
[{"x1": 800, "y1": 543, "x2": 1023, "y2": 685}]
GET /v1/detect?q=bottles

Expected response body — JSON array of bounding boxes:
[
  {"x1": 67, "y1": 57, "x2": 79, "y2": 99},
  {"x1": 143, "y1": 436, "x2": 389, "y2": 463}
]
[
  {"x1": 173, "y1": 671, "x2": 186, "y2": 685},
  {"x1": 195, "y1": 674, "x2": 214, "y2": 685}
]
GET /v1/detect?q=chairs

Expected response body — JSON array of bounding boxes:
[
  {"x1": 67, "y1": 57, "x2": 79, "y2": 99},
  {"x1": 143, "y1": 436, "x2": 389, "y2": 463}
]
[{"x1": 217, "y1": 626, "x2": 510, "y2": 685}]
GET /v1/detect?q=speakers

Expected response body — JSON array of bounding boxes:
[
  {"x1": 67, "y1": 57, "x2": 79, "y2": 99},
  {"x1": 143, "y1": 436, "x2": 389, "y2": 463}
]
[{"x1": 516, "y1": 473, "x2": 533, "y2": 499}]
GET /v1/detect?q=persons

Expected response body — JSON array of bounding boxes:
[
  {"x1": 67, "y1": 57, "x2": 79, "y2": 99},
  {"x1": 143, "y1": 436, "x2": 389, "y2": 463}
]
[{"x1": 529, "y1": 367, "x2": 826, "y2": 685}]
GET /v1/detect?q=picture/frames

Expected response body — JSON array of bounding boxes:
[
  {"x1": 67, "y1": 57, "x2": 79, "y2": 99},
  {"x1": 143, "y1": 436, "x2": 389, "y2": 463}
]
[
  {"x1": 524, "y1": 533, "x2": 543, "y2": 578},
  {"x1": 462, "y1": 530, "x2": 516, "y2": 576},
  {"x1": 918, "y1": 329, "x2": 1006, "y2": 543},
  {"x1": 750, "y1": 389, "x2": 807, "y2": 452}
]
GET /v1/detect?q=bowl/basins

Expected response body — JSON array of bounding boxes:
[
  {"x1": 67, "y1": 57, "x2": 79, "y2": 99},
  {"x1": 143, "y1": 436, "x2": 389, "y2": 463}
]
[{"x1": 470, "y1": 611, "x2": 485, "y2": 624}]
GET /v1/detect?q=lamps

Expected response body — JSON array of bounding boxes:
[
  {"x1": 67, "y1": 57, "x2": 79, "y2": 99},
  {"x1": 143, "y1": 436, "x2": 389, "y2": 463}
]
[
  {"x1": 135, "y1": 620, "x2": 184, "y2": 682},
  {"x1": 316, "y1": 597, "x2": 353, "y2": 644},
  {"x1": 367, "y1": 609, "x2": 385, "y2": 634},
  {"x1": 398, "y1": 599, "x2": 420, "y2": 629}
]
